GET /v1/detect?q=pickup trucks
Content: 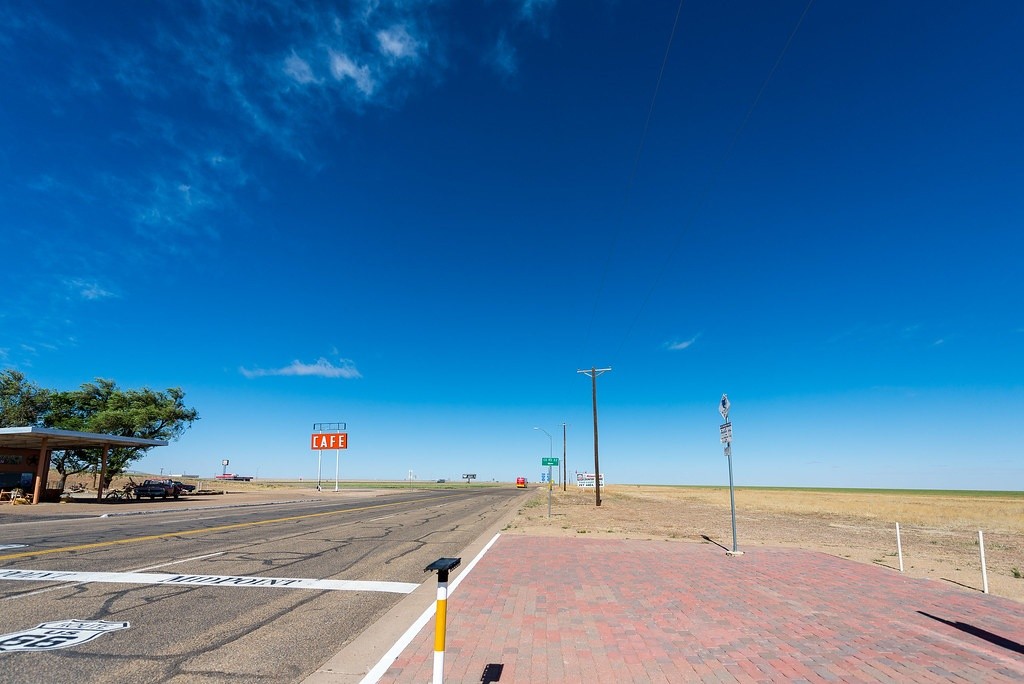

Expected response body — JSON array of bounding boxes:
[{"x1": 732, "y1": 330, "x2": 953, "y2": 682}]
[{"x1": 135, "y1": 478, "x2": 195, "y2": 502}]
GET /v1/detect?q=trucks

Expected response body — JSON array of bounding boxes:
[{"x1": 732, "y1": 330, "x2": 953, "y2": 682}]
[{"x1": 516, "y1": 477, "x2": 527, "y2": 488}]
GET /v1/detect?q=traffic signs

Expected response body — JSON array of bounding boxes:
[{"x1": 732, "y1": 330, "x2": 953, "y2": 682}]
[
  {"x1": 311, "y1": 433, "x2": 346, "y2": 449},
  {"x1": 542, "y1": 458, "x2": 558, "y2": 466}
]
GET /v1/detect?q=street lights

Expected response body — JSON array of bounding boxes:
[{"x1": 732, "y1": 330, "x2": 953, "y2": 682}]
[{"x1": 534, "y1": 427, "x2": 553, "y2": 520}]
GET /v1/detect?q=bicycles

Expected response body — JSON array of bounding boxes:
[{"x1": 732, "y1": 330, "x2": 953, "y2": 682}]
[{"x1": 105, "y1": 486, "x2": 134, "y2": 503}]
